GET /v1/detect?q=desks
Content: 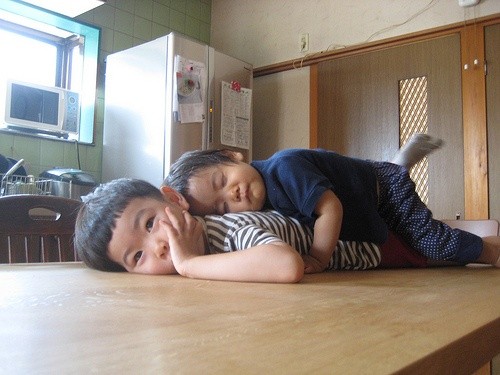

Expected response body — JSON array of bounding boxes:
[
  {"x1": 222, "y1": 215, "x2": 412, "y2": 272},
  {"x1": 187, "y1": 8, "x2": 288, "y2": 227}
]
[{"x1": 0, "y1": 261, "x2": 500, "y2": 375}]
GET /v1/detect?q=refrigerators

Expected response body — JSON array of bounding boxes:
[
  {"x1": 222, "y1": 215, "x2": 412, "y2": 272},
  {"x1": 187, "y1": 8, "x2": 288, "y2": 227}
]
[{"x1": 98, "y1": 31, "x2": 254, "y2": 189}]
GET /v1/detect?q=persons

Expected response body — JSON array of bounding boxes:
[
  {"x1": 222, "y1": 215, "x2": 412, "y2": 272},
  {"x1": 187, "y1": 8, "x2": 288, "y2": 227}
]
[
  {"x1": 161, "y1": 148, "x2": 500, "y2": 273},
  {"x1": 75, "y1": 133, "x2": 444, "y2": 283}
]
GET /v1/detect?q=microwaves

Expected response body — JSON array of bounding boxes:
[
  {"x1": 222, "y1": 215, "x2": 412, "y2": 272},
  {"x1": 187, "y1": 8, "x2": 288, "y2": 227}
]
[{"x1": 5, "y1": 79, "x2": 79, "y2": 137}]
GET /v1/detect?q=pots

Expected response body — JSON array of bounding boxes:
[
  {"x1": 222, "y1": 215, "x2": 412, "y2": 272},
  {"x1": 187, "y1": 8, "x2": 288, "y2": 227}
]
[{"x1": 38, "y1": 168, "x2": 99, "y2": 202}]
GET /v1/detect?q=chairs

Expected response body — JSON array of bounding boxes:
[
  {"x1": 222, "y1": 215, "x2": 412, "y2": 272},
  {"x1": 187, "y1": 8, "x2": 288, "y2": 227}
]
[{"x1": 0, "y1": 194, "x2": 83, "y2": 266}]
